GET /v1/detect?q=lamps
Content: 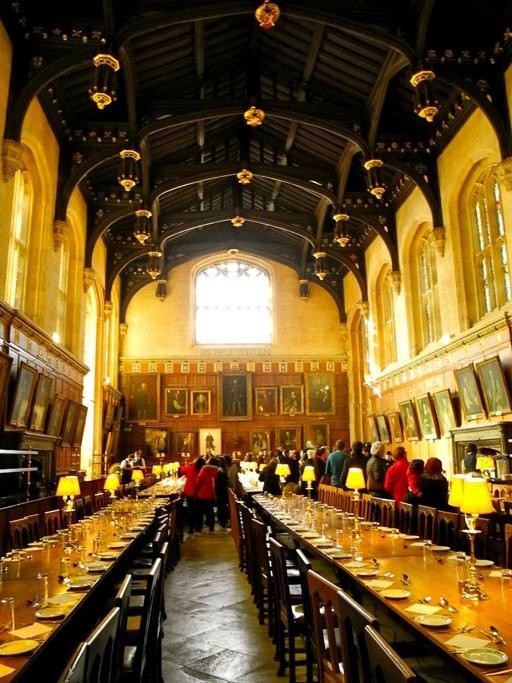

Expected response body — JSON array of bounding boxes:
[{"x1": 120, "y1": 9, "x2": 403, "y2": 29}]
[
  {"x1": 131, "y1": 470, "x2": 144, "y2": 502},
  {"x1": 301, "y1": 466, "x2": 316, "y2": 509},
  {"x1": 275, "y1": 462, "x2": 291, "y2": 500},
  {"x1": 447, "y1": 475, "x2": 497, "y2": 602},
  {"x1": 152, "y1": 461, "x2": 180, "y2": 479},
  {"x1": 239, "y1": 461, "x2": 268, "y2": 473},
  {"x1": 344, "y1": 468, "x2": 366, "y2": 540}
]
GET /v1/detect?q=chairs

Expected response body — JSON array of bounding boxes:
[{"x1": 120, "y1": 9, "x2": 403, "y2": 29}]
[
  {"x1": 397, "y1": 502, "x2": 414, "y2": 534},
  {"x1": 336, "y1": 589, "x2": 381, "y2": 683},
  {"x1": 494, "y1": 523, "x2": 511, "y2": 568},
  {"x1": 417, "y1": 505, "x2": 436, "y2": 541},
  {"x1": 436, "y1": 510, "x2": 490, "y2": 559},
  {"x1": 308, "y1": 570, "x2": 343, "y2": 683},
  {"x1": 269, "y1": 536, "x2": 312, "y2": 683},
  {"x1": 314, "y1": 485, "x2": 397, "y2": 528},
  {"x1": 229, "y1": 488, "x2": 274, "y2": 624},
  {"x1": 363, "y1": 625, "x2": 419, "y2": 683},
  {"x1": 0, "y1": 478, "x2": 185, "y2": 683}
]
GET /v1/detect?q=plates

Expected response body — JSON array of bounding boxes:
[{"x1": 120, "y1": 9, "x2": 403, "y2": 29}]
[
  {"x1": 0, "y1": 495, "x2": 171, "y2": 678},
  {"x1": 248, "y1": 488, "x2": 512, "y2": 669}
]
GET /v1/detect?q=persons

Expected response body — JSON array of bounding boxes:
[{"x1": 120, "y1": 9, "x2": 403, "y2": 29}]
[
  {"x1": 205, "y1": 432, "x2": 216, "y2": 453},
  {"x1": 404, "y1": 406, "x2": 415, "y2": 439},
  {"x1": 486, "y1": 368, "x2": 503, "y2": 412},
  {"x1": 120, "y1": 454, "x2": 135, "y2": 469},
  {"x1": 171, "y1": 392, "x2": 185, "y2": 414},
  {"x1": 228, "y1": 379, "x2": 243, "y2": 415},
  {"x1": 463, "y1": 376, "x2": 482, "y2": 415},
  {"x1": 261, "y1": 393, "x2": 273, "y2": 413},
  {"x1": 130, "y1": 452, "x2": 146, "y2": 467},
  {"x1": 178, "y1": 439, "x2": 449, "y2": 538},
  {"x1": 130, "y1": 380, "x2": 151, "y2": 419},
  {"x1": 461, "y1": 442, "x2": 499, "y2": 475}
]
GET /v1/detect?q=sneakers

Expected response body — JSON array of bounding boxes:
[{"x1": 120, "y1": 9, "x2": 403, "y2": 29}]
[{"x1": 182, "y1": 526, "x2": 233, "y2": 545}]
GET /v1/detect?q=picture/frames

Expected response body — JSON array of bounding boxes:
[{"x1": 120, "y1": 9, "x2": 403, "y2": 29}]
[
  {"x1": 125, "y1": 361, "x2": 335, "y2": 456},
  {"x1": 0, "y1": 354, "x2": 88, "y2": 447},
  {"x1": 368, "y1": 355, "x2": 512, "y2": 444}
]
